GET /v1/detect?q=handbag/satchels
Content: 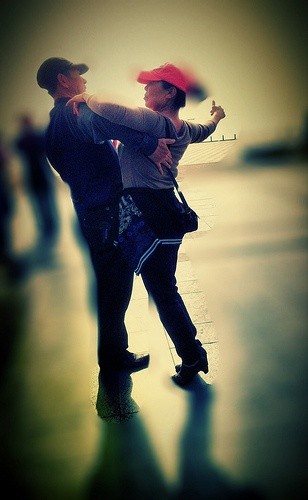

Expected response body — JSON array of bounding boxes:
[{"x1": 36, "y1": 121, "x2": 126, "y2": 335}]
[{"x1": 167, "y1": 190, "x2": 198, "y2": 234}]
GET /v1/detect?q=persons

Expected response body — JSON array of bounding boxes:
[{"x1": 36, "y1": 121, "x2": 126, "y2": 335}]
[
  {"x1": 35, "y1": 55, "x2": 175, "y2": 419},
  {"x1": 65, "y1": 62, "x2": 225, "y2": 386},
  {"x1": 10, "y1": 114, "x2": 62, "y2": 254}
]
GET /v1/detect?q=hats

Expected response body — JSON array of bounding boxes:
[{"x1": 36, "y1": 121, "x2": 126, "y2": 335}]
[
  {"x1": 137, "y1": 63, "x2": 188, "y2": 94},
  {"x1": 36, "y1": 56, "x2": 89, "y2": 89}
]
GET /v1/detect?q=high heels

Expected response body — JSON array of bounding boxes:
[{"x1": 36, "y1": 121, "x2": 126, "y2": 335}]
[{"x1": 172, "y1": 346, "x2": 208, "y2": 388}]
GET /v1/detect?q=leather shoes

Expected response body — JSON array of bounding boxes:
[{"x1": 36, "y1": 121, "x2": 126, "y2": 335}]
[{"x1": 100, "y1": 353, "x2": 149, "y2": 396}]
